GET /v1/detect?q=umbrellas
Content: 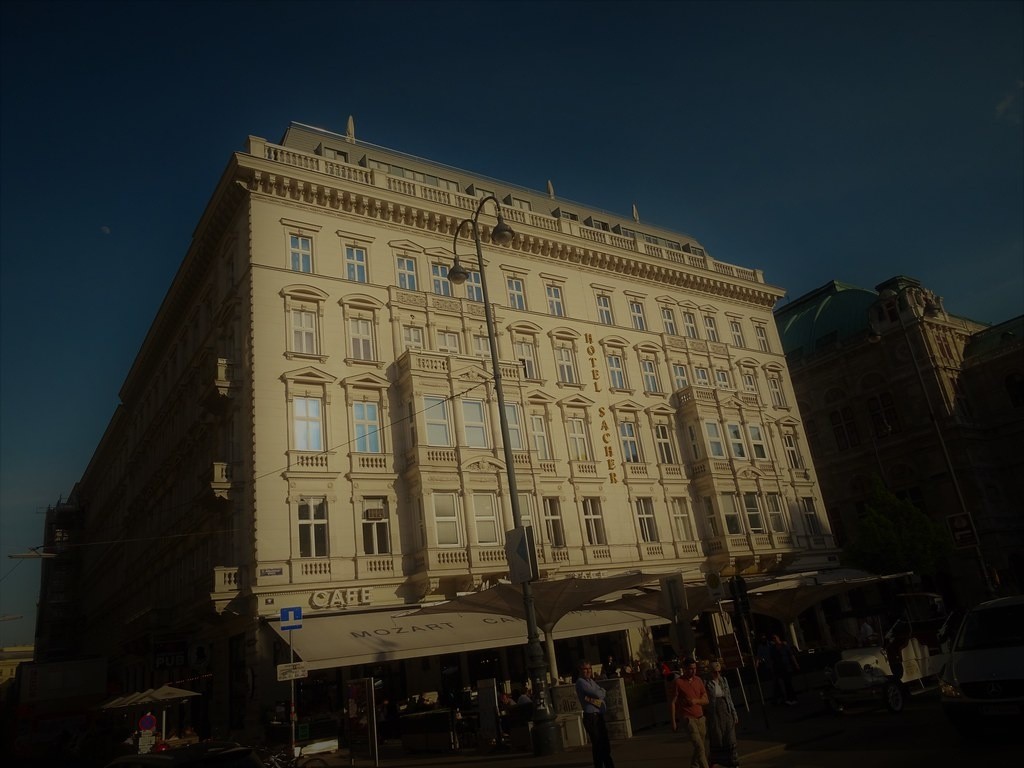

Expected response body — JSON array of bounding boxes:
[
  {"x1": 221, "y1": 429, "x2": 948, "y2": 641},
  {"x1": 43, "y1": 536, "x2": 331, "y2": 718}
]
[{"x1": 100, "y1": 685, "x2": 201, "y2": 741}]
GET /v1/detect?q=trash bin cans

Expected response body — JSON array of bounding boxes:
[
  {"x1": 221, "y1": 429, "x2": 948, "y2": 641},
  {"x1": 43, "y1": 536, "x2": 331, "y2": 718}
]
[
  {"x1": 508, "y1": 701, "x2": 538, "y2": 753},
  {"x1": 401, "y1": 709, "x2": 461, "y2": 753}
]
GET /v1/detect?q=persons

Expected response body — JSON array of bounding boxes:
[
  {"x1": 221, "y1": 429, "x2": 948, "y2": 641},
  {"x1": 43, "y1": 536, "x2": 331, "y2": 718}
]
[
  {"x1": 676, "y1": 656, "x2": 741, "y2": 768},
  {"x1": 601, "y1": 654, "x2": 631, "y2": 679},
  {"x1": 770, "y1": 633, "x2": 801, "y2": 673},
  {"x1": 575, "y1": 661, "x2": 615, "y2": 768},
  {"x1": 858, "y1": 618, "x2": 873, "y2": 643},
  {"x1": 165, "y1": 726, "x2": 196, "y2": 745}
]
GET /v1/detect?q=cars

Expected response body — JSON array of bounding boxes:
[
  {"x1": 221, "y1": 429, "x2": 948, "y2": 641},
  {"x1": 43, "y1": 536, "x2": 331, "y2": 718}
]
[
  {"x1": 938, "y1": 594, "x2": 1024, "y2": 743},
  {"x1": 821, "y1": 638, "x2": 934, "y2": 715}
]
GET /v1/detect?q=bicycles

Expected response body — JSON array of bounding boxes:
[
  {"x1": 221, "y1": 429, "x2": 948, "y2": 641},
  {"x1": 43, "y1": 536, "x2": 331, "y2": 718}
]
[{"x1": 263, "y1": 742, "x2": 331, "y2": 768}]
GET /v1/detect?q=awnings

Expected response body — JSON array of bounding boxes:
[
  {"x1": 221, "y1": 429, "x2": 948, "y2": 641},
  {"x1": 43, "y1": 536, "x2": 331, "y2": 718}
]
[
  {"x1": 268, "y1": 595, "x2": 672, "y2": 672},
  {"x1": 705, "y1": 568, "x2": 915, "y2": 616}
]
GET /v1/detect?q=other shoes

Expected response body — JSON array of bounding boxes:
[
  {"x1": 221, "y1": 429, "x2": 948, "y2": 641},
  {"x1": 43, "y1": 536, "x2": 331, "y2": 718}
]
[
  {"x1": 786, "y1": 701, "x2": 800, "y2": 705},
  {"x1": 708, "y1": 762, "x2": 718, "y2": 768}
]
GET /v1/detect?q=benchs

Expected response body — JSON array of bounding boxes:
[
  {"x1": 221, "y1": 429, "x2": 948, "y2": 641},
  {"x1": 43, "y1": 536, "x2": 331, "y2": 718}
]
[{"x1": 881, "y1": 630, "x2": 942, "y2": 664}]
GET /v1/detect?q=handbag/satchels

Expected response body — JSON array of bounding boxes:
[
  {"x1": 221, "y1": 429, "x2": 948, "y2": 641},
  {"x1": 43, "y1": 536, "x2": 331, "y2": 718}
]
[{"x1": 786, "y1": 645, "x2": 800, "y2": 675}]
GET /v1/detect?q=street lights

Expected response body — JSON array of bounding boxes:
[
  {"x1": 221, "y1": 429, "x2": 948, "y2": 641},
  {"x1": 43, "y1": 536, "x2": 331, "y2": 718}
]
[
  {"x1": 445, "y1": 195, "x2": 568, "y2": 760},
  {"x1": 865, "y1": 283, "x2": 981, "y2": 550}
]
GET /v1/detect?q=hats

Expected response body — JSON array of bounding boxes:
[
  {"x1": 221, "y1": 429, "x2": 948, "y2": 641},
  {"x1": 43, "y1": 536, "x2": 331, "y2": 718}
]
[{"x1": 710, "y1": 662, "x2": 722, "y2": 671}]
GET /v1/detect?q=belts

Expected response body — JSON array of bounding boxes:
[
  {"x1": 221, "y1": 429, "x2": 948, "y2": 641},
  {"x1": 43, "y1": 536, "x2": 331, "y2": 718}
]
[{"x1": 583, "y1": 713, "x2": 598, "y2": 716}]
